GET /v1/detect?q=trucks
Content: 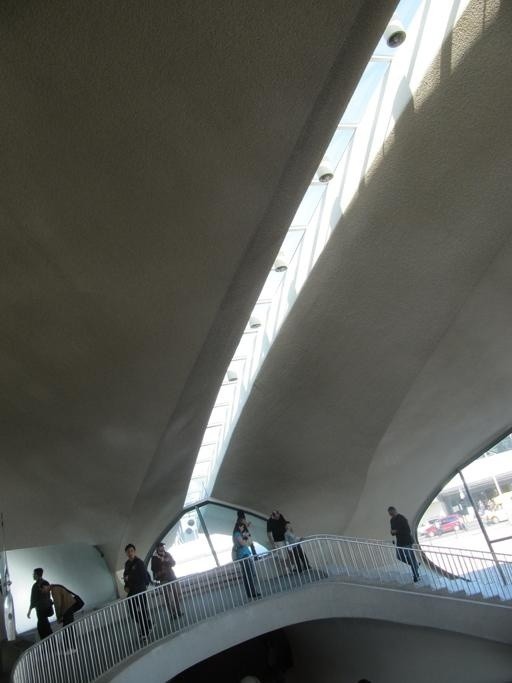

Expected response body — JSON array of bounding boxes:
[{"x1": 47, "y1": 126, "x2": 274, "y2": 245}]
[{"x1": 484, "y1": 491, "x2": 512, "y2": 524}]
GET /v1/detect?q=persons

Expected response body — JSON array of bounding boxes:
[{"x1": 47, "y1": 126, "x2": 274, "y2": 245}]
[
  {"x1": 234, "y1": 508, "x2": 260, "y2": 561},
  {"x1": 151, "y1": 543, "x2": 184, "y2": 621},
  {"x1": 38, "y1": 580, "x2": 79, "y2": 656},
  {"x1": 267, "y1": 511, "x2": 297, "y2": 576},
  {"x1": 124, "y1": 544, "x2": 156, "y2": 640},
  {"x1": 28, "y1": 569, "x2": 56, "y2": 653},
  {"x1": 388, "y1": 506, "x2": 421, "y2": 581},
  {"x1": 285, "y1": 521, "x2": 312, "y2": 574},
  {"x1": 233, "y1": 522, "x2": 262, "y2": 597}
]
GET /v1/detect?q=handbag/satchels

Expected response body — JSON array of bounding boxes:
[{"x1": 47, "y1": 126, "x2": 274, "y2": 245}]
[{"x1": 74, "y1": 596, "x2": 84, "y2": 611}]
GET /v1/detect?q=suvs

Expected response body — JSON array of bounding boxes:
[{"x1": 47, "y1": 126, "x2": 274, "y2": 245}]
[
  {"x1": 420, "y1": 517, "x2": 439, "y2": 535},
  {"x1": 426, "y1": 513, "x2": 464, "y2": 536}
]
